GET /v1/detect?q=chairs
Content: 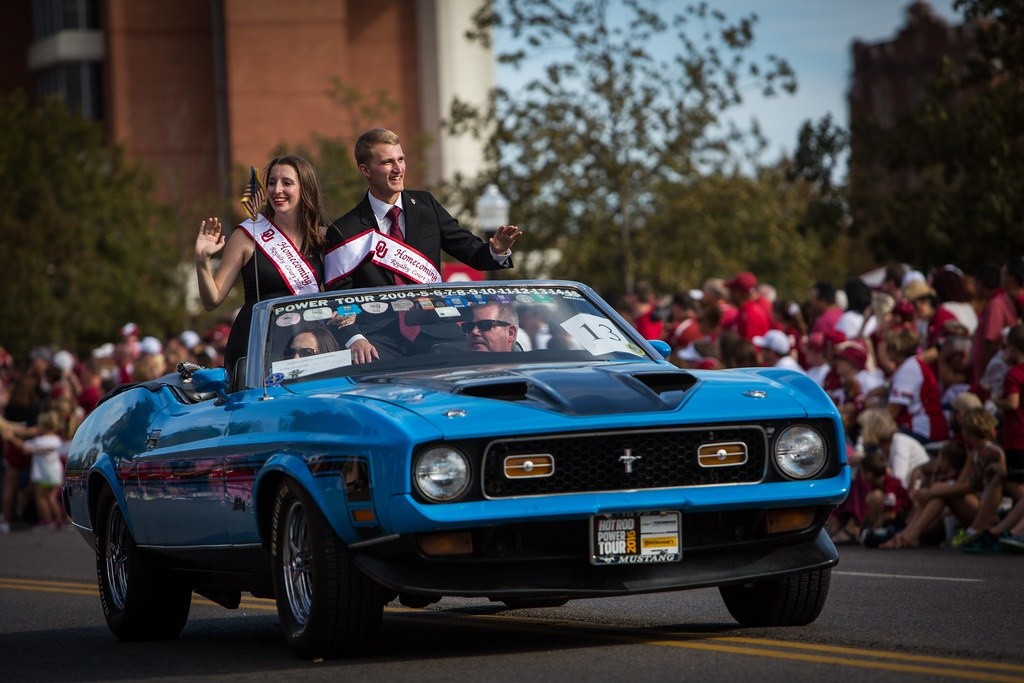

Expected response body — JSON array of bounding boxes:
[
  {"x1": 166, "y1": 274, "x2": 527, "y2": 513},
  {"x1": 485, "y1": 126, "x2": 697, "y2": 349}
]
[{"x1": 231, "y1": 353, "x2": 284, "y2": 392}]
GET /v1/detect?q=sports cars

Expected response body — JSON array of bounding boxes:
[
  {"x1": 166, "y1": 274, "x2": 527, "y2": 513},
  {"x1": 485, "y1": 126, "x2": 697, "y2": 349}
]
[{"x1": 59, "y1": 279, "x2": 851, "y2": 658}]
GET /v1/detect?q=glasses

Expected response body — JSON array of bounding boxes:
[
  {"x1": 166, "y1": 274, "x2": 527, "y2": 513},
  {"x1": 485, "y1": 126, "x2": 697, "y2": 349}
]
[
  {"x1": 934, "y1": 342, "x2": 945, "y2": 353},
  {"x1": 461, "y1": 319, "x2": 511, "y2": 335},
  {"x1": 284, "y1": 348, "x2": 316, "y2": 360}
]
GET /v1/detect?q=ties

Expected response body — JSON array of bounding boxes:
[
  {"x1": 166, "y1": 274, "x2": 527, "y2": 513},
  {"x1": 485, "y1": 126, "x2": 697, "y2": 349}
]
[{"x1": 386, "y1": 207, "x2": 420, "y2": 341}]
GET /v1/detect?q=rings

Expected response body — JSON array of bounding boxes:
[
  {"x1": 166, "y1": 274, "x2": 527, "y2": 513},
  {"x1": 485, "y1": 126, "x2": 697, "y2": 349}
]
[{"x1": 346, "y1": 320, "x2": 347, "y2": 324}]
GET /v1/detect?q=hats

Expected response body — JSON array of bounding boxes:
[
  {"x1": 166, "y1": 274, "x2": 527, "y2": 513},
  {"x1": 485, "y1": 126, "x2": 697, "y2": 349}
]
[
  {"x1": 752, "y1": 329, "x2": 790, "y2": 353},
  {"x1": 952, "y1": 392, "x2": 983, "y2": 412},
  {"x1": 835, "y1": 339, "x2": 869, "y2": 369},
  {"x1": 725, "y1": 273, "x2": 757, "y2": 292}
]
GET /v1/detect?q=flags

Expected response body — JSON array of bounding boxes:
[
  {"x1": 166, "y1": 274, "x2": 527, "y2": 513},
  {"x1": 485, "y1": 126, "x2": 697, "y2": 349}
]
[{"x1": 241, "y1": 168, "x2": 268, "y2": 221}]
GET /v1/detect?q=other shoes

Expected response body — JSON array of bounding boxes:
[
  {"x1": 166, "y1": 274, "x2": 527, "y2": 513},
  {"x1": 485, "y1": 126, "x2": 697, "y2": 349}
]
[{"x1": 831, "y1": 521, "x2": 1024, "y2": 552}]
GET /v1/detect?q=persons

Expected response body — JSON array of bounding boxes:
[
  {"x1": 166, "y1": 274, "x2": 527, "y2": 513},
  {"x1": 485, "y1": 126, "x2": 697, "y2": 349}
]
[
  {"x1": 282, "y1": 325, "x2": 339, "y2": 360},
  {"x1": 195, "y1": 157, "x2": 356, "y2": 377},
  {"x1": 0, "y1": 315, "x2": 233, "y2": 531},
  {"x1": 323, "y1": 128, "x2": 522, "y2": 365},
  {"x1": 461, "y1": 300, "x2": 519, "y2": 353},
  {"x1": 619, "y1": 261, "x2": 1024, "y2": 553}
]
